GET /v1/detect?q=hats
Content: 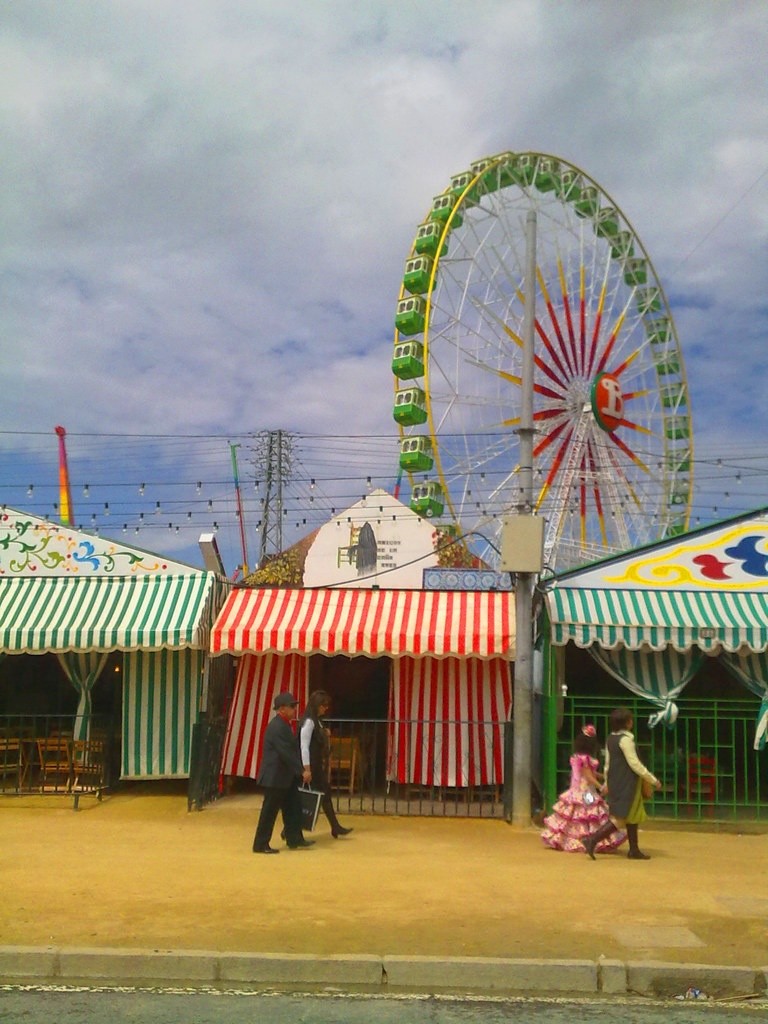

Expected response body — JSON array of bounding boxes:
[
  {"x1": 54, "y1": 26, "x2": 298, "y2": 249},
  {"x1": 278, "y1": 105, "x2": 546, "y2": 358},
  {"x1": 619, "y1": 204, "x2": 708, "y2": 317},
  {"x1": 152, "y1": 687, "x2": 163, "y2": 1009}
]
[
  {"x1": 272, "y1": 692, "x2": 300, "y2": 710},
  {"x1": 582, "y1": 724, "x2": 597, "y2": 738}
]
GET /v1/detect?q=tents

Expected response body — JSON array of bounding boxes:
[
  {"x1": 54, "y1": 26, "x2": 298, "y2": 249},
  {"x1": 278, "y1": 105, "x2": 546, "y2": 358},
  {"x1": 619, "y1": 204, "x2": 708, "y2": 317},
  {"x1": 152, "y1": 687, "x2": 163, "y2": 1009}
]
[{"x1": 0, "y1": 505, "x2": 768, "y2": 821}]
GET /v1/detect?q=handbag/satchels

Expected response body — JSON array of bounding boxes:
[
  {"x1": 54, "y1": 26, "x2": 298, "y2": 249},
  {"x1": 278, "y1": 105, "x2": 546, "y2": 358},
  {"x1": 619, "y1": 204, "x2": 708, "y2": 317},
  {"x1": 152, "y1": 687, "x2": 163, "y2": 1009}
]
[{"x1": 296, "y1": 782, "x2": 325, "y2": 832}]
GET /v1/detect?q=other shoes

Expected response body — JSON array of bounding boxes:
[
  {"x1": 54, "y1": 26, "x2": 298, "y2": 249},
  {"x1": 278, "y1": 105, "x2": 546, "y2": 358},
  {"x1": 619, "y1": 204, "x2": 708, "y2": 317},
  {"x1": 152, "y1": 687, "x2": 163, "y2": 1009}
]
[
  {"x1": 253, "y1": 845, "x2": 279, "y2": 854},
  {"x1": 287, "y1": 838, "x2": 315, "y2": 848}
]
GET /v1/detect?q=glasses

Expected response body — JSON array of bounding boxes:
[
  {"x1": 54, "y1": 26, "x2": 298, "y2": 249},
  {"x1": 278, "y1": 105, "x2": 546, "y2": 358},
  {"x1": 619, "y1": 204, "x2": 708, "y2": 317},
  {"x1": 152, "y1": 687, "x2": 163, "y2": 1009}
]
[
  {"x1": 286, "y1": 704, "x2": 296, "y2": 709},
  {"x1": 321, "y1": 703, "x2": 328, "y2": 707}
]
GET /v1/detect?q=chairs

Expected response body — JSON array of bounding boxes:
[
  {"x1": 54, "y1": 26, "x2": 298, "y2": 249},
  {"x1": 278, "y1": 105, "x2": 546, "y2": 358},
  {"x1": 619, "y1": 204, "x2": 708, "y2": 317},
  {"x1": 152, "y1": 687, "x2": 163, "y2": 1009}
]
[
  {"x1": 35, "y1": 738, "x2": 71, "y2": 794},
  {"x1": 678, "y1": 756, "x2": 715, "y2": 816},
  {"x1": 649, "y1": 753, "x2": 674, "y2": 793},
  {"x1": 71, "y1": 741, "x2": 107, "y2": 799},
  {"x1": 0, "y1": 736, "x2": 26, "y2": 791},
  {"x1": 327, "y1": 737, "x2": 359, "y2": 796}
]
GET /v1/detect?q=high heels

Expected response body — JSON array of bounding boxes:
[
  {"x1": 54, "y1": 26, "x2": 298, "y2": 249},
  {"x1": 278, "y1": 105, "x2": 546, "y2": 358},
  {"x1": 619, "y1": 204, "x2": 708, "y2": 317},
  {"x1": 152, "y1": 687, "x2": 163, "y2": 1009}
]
[
  {"x1": 281, "y1": 827, "x2": 287, "y2": 841},
  {"x1": 331, "y1": 822, "x2": 353, "y2": 838}
]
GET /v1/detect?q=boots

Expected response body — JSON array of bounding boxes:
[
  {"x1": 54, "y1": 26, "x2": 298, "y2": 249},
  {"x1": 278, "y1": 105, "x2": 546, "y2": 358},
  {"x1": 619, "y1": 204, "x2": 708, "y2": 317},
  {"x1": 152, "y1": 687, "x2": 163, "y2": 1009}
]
[
  {"x1": 626, "y1": 824, "x2": 651, "y2": 860},
  {"x1": 581, "y1": 819, "x2": 617, "y2": 860}
]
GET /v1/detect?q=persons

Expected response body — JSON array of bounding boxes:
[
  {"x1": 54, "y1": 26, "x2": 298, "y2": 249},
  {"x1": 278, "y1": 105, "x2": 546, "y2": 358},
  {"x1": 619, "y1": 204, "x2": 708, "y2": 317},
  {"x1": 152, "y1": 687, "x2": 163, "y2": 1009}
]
[
  {"x1": 582, "y1": 709, "x2": 662, "y2": 861},
  {"x1": 253, "y1": 692, "x2": 316, "y2": 854},
  {"x1": 540, "y1": 725, "x2": 627, "y2": 854},
  {"x1": 280, "y1": 690, "x2": 353, "y2": 841}
]
